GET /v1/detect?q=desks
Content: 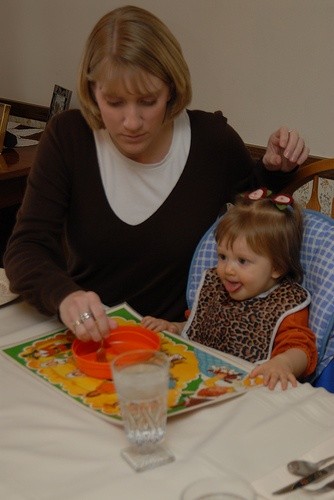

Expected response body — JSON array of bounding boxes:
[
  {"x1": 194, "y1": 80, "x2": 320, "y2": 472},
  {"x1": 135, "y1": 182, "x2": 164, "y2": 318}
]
[{"x1": 0, "y1": 300, "x2": 334, "y2": 500}]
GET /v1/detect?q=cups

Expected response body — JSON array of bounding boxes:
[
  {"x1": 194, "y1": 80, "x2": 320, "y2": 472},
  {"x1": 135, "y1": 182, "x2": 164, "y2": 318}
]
[{"x1": 180, "y1": 473, "x2": 257, "y2": 500}]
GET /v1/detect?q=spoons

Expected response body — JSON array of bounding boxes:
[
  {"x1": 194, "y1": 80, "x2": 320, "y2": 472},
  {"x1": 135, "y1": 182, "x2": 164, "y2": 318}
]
[{"x1": 287, "y1": 455, "x2": 334, "y2": 477}]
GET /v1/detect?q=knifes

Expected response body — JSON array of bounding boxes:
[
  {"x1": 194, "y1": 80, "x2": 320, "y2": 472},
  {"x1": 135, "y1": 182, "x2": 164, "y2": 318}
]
[{"x1": 272, "y1": 462, "x2": 334, "y2": 494}]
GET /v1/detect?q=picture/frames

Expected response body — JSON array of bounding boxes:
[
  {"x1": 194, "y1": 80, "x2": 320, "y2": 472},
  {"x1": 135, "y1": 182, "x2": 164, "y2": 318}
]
[{"x1": 47, "y1": 84, "x2": 73, "y2": 118}]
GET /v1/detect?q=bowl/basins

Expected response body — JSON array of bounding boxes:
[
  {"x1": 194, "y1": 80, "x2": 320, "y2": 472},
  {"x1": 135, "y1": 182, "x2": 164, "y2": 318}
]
[{"x1": 70, "y1": 323, "x2": 162, "y2": 380}]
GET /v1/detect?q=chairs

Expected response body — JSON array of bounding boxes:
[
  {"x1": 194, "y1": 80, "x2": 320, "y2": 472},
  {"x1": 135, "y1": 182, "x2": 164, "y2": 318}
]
[{"x1": 186, "y1": 157, "x2": 334, "y2": 393}]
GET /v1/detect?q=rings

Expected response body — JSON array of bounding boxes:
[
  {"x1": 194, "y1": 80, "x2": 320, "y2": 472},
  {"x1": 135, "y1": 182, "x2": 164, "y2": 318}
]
[
  {"x1": 81, "y1": 312, "x2": 93, "y2": 322},
  {"x1": 73, "y1": 320, "x2": 84, "y2": 328}
]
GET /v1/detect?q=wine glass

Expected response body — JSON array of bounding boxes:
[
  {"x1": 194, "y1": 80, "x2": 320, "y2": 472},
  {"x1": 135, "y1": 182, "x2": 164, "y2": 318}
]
[{"x1": 108, "y1": 349, "x2": 175, "y2": 474}]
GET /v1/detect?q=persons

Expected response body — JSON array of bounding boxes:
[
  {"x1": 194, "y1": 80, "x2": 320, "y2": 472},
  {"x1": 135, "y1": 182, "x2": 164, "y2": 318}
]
[
  {"x1": 5, "y1": 6, "x2": 309, "y2": 344},
  {"x1": 140, "y1": 189, "x2": 317, "y2": 392}
]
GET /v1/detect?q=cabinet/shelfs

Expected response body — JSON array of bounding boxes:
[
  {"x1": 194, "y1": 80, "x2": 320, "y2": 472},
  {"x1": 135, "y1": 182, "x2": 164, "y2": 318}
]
[{"x1": 0, "y1": 121, "x2": 44, "y2": 208}]
